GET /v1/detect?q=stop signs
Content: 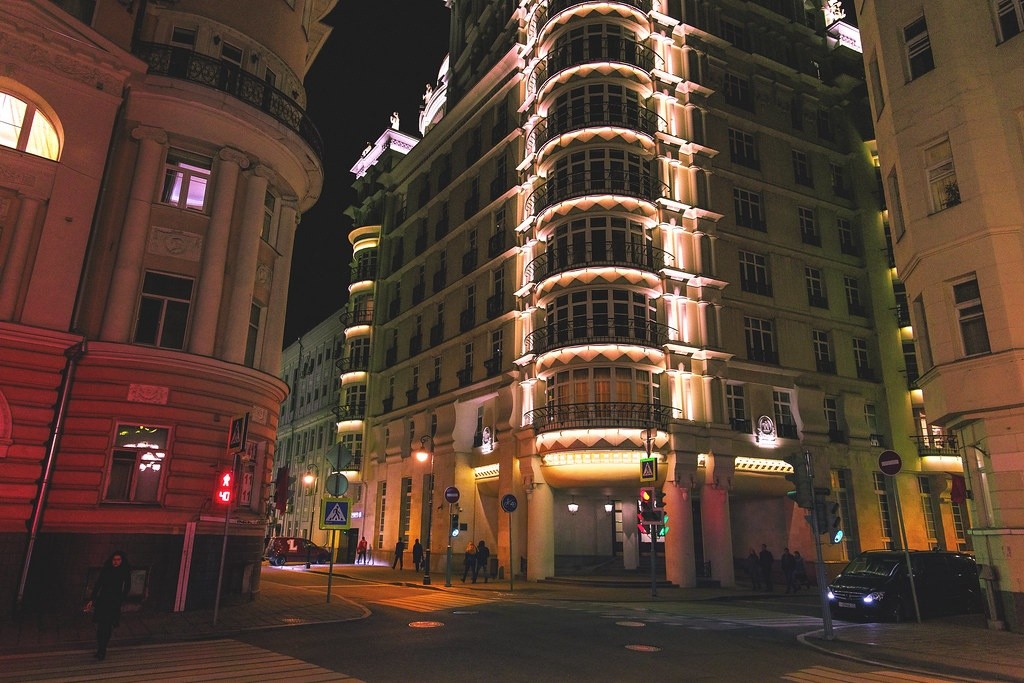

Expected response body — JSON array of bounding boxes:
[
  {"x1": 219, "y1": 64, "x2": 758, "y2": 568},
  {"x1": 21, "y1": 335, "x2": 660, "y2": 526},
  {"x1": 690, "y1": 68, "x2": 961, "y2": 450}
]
[
  {"x1": 878, "y1": 450, "x2": 903, "y2": 476},
  {"x1": 444, "y1": 487, "x2": 460, "y2": 504}
]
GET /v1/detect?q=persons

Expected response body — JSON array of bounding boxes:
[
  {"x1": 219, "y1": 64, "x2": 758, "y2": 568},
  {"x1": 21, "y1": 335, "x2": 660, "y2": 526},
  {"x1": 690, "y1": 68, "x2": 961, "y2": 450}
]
[
  {"x1": 357, "y1": 537, "x2": 367, "y2": 565},
  {"x1": 263, "y1": 535, "x2": 270, "y2": 555},
  {"x1": 85, "y1": 550, "x2": 131, "y2": 660},
  {"x1": 471, "y1": 540, "x2": 489, "y2": 583},
  {"x1": 746, "y1": 547, "x2": 762, "y2": 591},
  {"x1": 392, "y1": 537, "x2": 404, "y2": 570},
  {"x1": 793, "y1": 551, "x2": 810, "y2": 590},
  {"x1": 413, "y1": 539, "x2": 423, "y2": 573},
  {"x1": 781, "y1": 548, "x2": 798, "y2": 594},
  {"x1": 759, "y1": 544, "x2": 774, "y2": 592},
  {"x1": 460, "y1": 541, "x2": 477, "y2": 583}
]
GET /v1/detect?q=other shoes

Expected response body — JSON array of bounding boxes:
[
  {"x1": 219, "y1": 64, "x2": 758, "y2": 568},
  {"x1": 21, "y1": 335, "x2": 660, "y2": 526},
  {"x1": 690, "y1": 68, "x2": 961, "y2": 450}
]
[
  {"x1": 95, "y1": 648, "x2": 105, "y2": 660},
  {"x1": 399, "y1": 568, "x2": 402, "y2": 571},
  {"x1": 391, "y1": 566, "x2": 395, "y2": 570},
  {"x1": 460, "y1": 578, "x2": 465, "y2": 583}
]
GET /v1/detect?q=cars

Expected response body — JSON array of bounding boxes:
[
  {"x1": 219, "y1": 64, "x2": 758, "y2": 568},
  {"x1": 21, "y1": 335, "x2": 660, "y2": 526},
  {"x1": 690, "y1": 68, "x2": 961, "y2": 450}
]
[{"x1": 264, "y1": 537, "x2": 329, "y2": 566}]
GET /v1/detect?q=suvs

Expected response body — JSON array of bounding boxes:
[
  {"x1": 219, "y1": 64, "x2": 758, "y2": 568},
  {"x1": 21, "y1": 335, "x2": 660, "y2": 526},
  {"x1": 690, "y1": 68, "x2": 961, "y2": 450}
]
[{"x1": 827, "y1": 548, "x2": 981, "y2": 623}]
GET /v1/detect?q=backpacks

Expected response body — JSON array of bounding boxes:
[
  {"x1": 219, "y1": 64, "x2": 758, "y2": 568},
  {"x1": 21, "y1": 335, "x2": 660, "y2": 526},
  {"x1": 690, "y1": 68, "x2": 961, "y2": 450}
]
[{"x1": 467, "y1": 544, "x2": 476, "y2": 555}]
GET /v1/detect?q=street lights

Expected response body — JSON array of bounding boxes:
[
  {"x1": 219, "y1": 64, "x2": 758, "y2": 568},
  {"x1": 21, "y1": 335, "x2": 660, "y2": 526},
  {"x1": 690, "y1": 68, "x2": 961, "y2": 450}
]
[{"x1": 410, "y1": 435, "x2": 435, "y2": 585}]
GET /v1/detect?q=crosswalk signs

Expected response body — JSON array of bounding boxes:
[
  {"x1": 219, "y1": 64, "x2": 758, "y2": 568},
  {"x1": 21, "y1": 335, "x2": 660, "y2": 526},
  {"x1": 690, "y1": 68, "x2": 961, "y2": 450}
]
[{"x1": 324, "y1": 501, "x2": 348, "y2": 525}]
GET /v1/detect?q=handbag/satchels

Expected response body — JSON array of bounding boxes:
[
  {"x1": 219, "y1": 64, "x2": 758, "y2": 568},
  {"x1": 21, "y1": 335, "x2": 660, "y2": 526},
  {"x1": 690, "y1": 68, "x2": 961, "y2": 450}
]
[
  {"x1": 357, "y1": 548, "x2": 362, "y2": 554},
  {"x1": 420, "y1": 560, "x2": 425, "y2": 569}
]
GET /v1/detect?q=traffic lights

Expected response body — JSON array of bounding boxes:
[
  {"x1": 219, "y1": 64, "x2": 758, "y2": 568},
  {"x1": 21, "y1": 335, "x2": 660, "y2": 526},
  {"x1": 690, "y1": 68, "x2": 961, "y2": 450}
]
[
  {"x1": 782, "y1": 451, "x2": 812, "y2": 508},
  {"x1": 217, "y1": 473, "x2": 234, "y2": 503}
]
